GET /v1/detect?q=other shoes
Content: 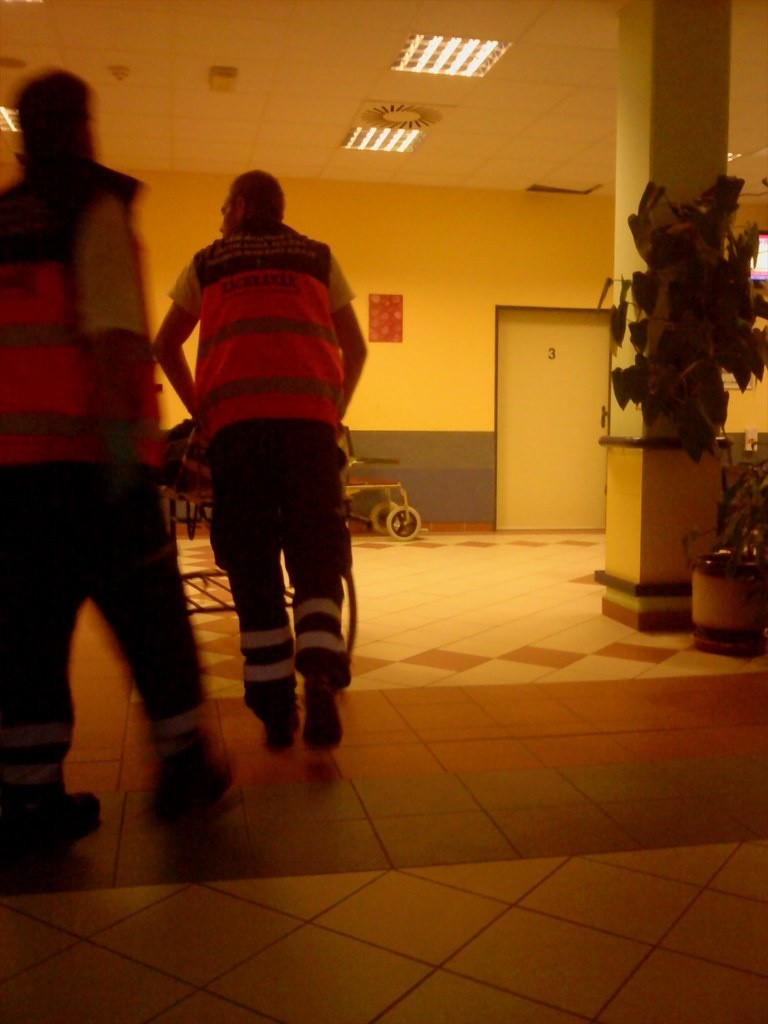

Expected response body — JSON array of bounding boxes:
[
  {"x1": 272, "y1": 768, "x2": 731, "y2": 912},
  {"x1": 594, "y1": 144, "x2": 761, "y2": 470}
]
[
  {"x1": 0, "y1": 788, "x2": 98, "y2": 858},
  {"x1": 304, "y1": 675, "x2": 343, "y2": 752},
  {"x1": 160, "y1": 755, "x2": 234, "y2": 824},
  {"x1": 263, "y1": 697, "x2": 299, "y2": 748}
]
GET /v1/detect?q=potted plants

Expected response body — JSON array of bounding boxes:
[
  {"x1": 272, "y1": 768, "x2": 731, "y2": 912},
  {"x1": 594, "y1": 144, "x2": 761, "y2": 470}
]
[{"x1": 677, "y1": 458, "x2": 768, "y2": 657}]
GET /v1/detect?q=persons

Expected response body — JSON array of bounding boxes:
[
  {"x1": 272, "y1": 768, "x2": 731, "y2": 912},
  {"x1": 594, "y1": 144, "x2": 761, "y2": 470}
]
[
  {"x1": 152, "y1": 169, "x2": 368, "y2": 755},
  {"x1": 0, "y1": 70, "x2": 233, "y2": 839}
]
